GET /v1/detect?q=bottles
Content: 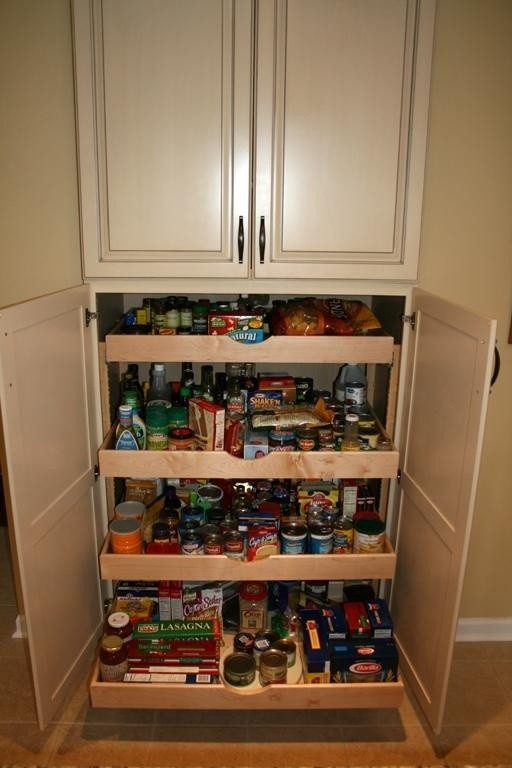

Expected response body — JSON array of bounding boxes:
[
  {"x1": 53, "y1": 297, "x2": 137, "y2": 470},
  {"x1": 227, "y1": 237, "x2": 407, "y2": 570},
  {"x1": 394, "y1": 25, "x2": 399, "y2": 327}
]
[
  {"x1": 113, "y1": 364, "x2": 145, "y2": 448},
  {"x1": 342, "y1": 414, "x2": 360, "y2": 451},
  {"x1": 237, "y1": 581, "x2": 268, "y2": 634},
  {"x1": 145, "y1": 361, "x2": 227, "y2": 405},
  {"x1": 164, "y1": 486, "x2": 182, "y2": 518},
  {"x1": 226, "y1": 376, "x2": 245, "y2": 413},
  {"x1": 98, "y1": 612, "x2": 133, "y2": 683},
  {"x1": 136, "y1": 294, "x2": 272, "y2": 339},
  {"x1": 226, "y1": 362, "x2": 259, "y2": 390},
  {"x1": 230, "y1": 484, "x2": 252, "y2": 520}
]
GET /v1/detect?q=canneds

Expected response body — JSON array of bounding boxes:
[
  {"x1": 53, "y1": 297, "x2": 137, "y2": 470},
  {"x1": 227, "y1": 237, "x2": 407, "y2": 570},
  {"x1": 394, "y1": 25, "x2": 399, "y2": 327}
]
[
  {"x1": 280, "y1": 505, "x2": 384, "y2": 554},
  {"x1": 269, "y1": 389, "x2": 394, "y2": 450},
  {"x1": 224, "y1": 629, "x2": 296, "y2": 686},
  {"x1": 160, "y1": 504, "x2": 251, "y2": 555},
  {"x1": 108, "y1": 612, "x2": 133, "y2": 639},
  {"x1": 110, "y1": 501, "x2": 146, "y2": 554},
  {"x1": 169, "y1": 428, "x2": 195, "y2": 451},
  {"x1": 99, "y1": 636, "x2": 128, "y2": 680}
]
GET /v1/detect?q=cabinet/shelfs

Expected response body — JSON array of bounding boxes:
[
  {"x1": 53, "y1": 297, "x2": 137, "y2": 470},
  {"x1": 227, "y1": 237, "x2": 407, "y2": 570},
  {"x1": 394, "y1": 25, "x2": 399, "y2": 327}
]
[
  {"x1": 2, "y1": 282, "x2": 500, "y2": 734},
  {"x1": 69, "y1": 2, "x2": 437, "y2": 284}
]
